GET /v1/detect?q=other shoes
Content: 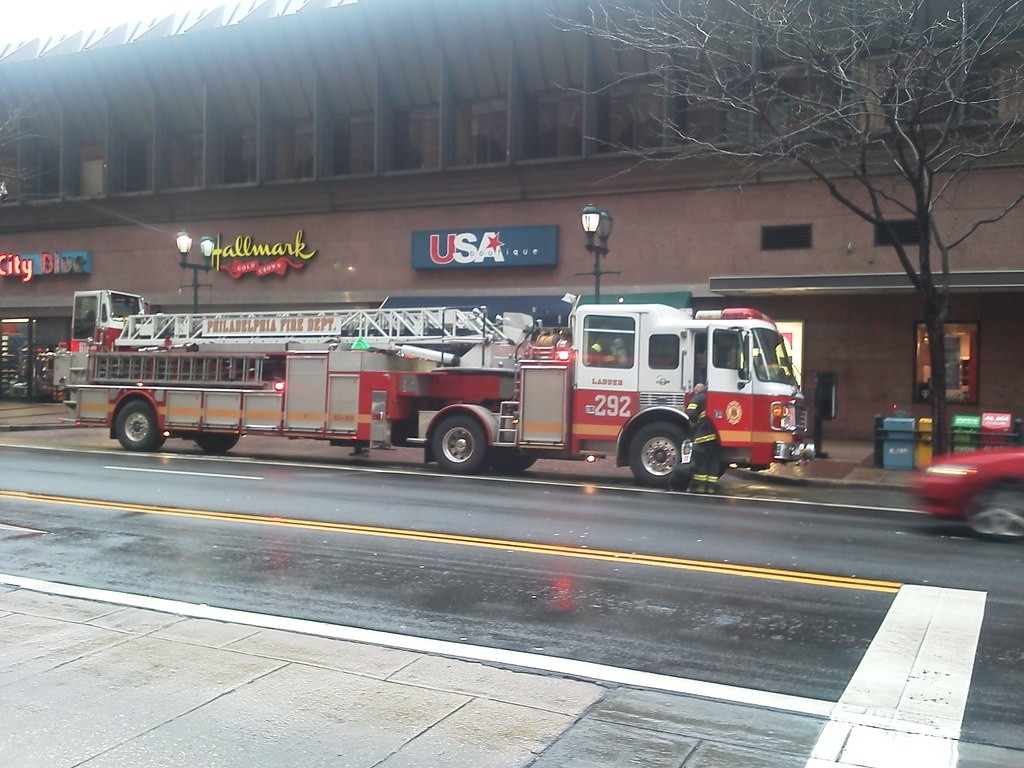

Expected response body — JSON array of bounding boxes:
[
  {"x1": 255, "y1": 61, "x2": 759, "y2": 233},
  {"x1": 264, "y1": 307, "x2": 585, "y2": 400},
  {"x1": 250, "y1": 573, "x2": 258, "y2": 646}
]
[
  {"x1": 705, "y1": 488, "x2": 714, "y2": 494},
  {"x1": 686, "y1": 488, "x2": 705, "y2": 493}
]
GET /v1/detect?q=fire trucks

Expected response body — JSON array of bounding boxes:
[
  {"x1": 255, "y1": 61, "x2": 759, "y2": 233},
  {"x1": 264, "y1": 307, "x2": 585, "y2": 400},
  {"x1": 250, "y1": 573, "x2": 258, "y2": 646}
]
[{"x1": 46, "y1": 289, "x2": 819, "y2": 492}]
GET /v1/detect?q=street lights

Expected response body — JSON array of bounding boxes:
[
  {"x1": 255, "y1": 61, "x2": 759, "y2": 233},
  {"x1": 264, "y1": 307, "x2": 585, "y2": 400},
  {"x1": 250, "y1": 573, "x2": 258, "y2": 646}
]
[
  {"x1": 574, "y1": 203, "x2": 622, "y2": 304},
  {"x1": 171, "y1": 230, "x2": 217, "y2": 314}
]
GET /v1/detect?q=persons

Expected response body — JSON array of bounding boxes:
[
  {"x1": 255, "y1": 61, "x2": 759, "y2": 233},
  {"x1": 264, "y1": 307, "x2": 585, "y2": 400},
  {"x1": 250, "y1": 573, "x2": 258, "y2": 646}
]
[
  {"x1": 590, "y1": 323, "x2": 618, "y2": 367},
  {"x1": 684, "y1": 382, "x2": 721, "y2": 493}
]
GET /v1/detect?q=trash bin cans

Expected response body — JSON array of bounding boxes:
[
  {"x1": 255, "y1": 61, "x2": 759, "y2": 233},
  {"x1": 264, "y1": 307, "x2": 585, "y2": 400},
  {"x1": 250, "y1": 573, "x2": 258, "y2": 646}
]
[
  {"x1": 981, "y1": 411, "x2": 1013, "y2": 448},
  {"x1": 916, "y1": 418, "x2": 933, "y2": 470},
  {"x1": 882, "y1": 416, "x2": 915, "y2": 473},
  {"x1": 950, "y1": 414, "x2": 980, "y2": 454},
  {"x1": 1015, "y1": 418, "x2": 1024, "y2": 447}
]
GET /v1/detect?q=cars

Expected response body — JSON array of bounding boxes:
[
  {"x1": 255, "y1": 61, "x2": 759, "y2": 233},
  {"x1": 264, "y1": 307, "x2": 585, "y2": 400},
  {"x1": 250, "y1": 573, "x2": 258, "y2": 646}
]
[{"x1": 907, "y1": 443, "x2": 1024, "y2": 543}]
